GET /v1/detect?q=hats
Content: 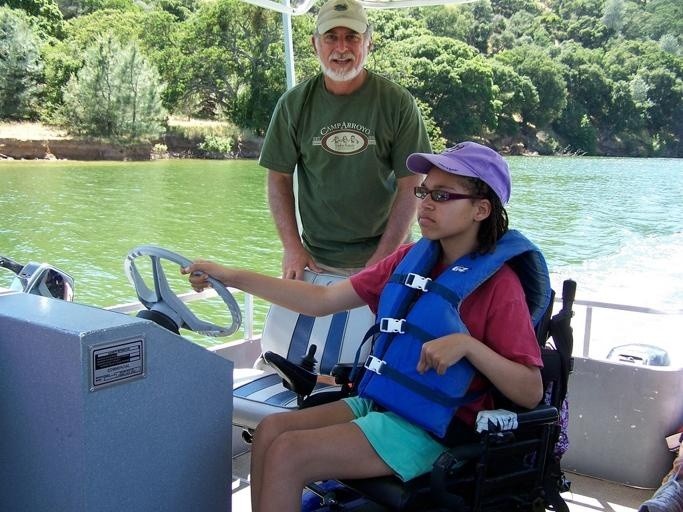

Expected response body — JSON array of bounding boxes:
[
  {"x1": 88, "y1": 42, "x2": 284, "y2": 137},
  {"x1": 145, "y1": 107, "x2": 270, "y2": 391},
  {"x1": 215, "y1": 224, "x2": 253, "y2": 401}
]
[
  {"x1": 316, "y1": 0, "x2": 369, "y2": 33},
  {"x1": 406, "y1": 140, "x2": 512, "y2": 207}
]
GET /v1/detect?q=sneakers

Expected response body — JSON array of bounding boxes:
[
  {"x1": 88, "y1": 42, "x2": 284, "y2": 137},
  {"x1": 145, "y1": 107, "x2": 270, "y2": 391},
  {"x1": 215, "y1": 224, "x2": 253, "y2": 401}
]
[{"x1": 639, "y1": 473, "x2": 682, "y2": 511}]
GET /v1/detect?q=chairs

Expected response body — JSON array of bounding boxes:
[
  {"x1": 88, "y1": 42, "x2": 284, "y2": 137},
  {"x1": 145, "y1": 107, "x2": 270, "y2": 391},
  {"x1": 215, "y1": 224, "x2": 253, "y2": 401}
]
[
  {"x1": 263, "y1": 279, "x2": 577, "y2": 512},
  {"x1": 232, "y1": 269, "x2": 379, "y2": 445}
]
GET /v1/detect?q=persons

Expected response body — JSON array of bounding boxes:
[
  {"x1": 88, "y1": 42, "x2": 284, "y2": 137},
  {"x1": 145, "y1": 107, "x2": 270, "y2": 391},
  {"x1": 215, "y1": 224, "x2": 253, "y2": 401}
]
[
  {"x1": 179, "y1": 140, "x2": 547, "y2": 512},
  {"x1": 257, "y1": 1, "x2": 433, "y2": 281}
]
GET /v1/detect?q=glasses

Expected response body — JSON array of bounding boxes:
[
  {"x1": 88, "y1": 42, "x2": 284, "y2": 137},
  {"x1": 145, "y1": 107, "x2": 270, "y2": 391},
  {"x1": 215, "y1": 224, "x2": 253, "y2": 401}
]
[{"x1": 414, "y1": 186, "x2": 481, "y2": 205}]
